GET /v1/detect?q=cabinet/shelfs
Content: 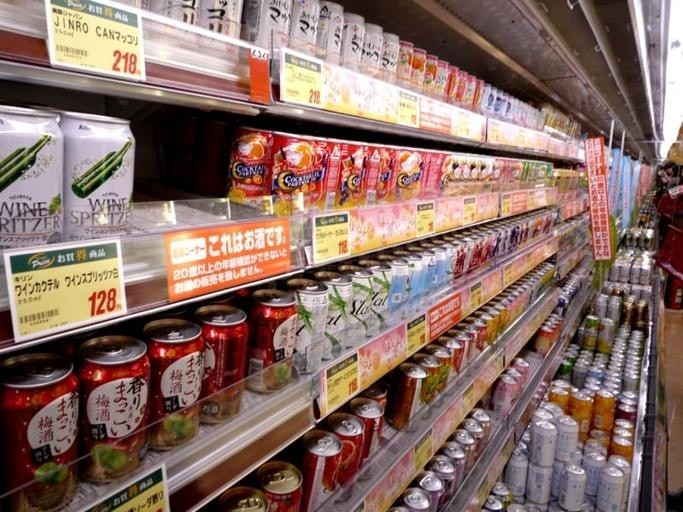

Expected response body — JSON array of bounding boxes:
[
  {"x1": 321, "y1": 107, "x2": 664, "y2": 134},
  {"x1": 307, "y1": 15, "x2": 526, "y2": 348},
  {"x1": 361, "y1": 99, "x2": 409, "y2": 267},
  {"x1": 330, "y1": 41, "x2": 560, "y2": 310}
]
[{"x1": 0, "y1": 0, "x2": 671, "y2": 512}]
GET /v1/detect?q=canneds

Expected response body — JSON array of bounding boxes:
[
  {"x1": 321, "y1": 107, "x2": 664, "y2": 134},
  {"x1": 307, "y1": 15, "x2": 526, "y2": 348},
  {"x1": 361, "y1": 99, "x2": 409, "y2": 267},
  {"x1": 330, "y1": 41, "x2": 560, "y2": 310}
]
[{"x1": 1, "y1": 0, "x2": 657, "y2": 512}]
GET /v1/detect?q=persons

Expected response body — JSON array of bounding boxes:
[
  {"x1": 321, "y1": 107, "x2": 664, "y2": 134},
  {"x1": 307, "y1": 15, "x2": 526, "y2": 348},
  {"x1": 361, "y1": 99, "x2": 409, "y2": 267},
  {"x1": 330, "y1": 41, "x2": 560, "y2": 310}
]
[{"x1": 652, "y1": 158, "x2": 683, "y2": 309}]
